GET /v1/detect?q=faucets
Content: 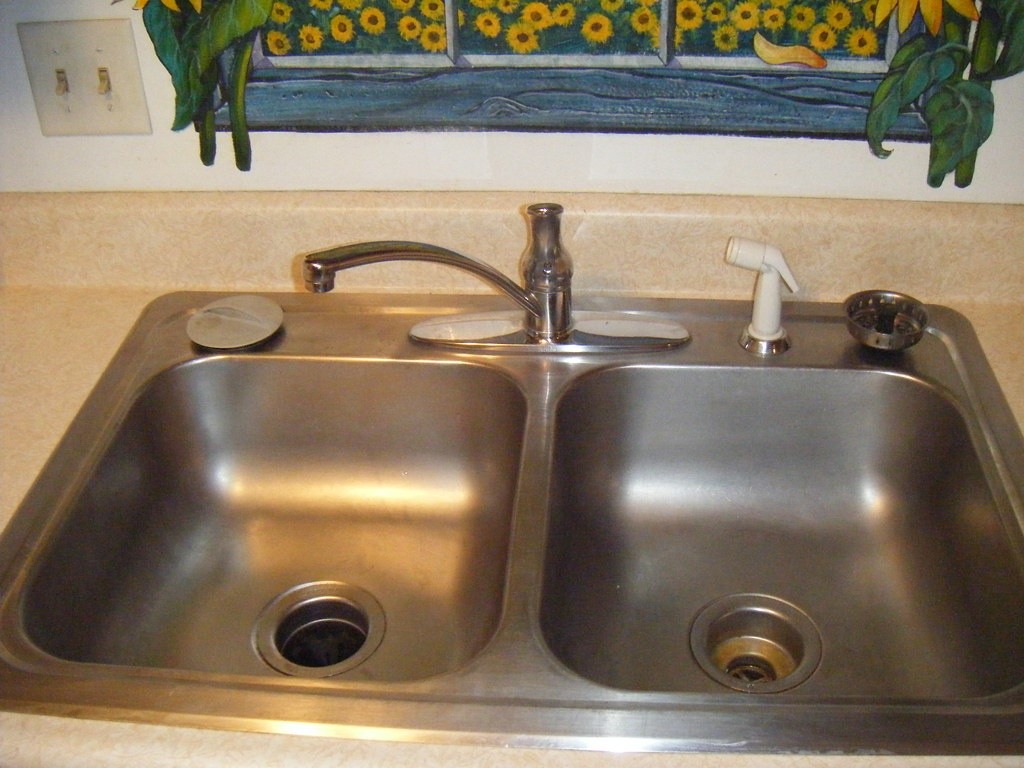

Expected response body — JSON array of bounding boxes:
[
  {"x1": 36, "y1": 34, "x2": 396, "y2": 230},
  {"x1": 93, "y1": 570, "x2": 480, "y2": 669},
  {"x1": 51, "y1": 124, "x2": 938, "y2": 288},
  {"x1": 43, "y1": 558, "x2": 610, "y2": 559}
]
[{"x1": 302, "y1": 202, "x2": 691, "y2": 357}]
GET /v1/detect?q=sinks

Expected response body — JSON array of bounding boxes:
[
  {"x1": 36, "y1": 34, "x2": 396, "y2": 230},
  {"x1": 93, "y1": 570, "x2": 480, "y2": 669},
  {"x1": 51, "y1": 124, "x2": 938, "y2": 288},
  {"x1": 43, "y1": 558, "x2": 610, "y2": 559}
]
[
  {"x1": 537, "y1": 294, "x2": 1024, "y2": 757},
  {"x1": 2, "y1": 290, "x2": 527, "y2": 735}
]
